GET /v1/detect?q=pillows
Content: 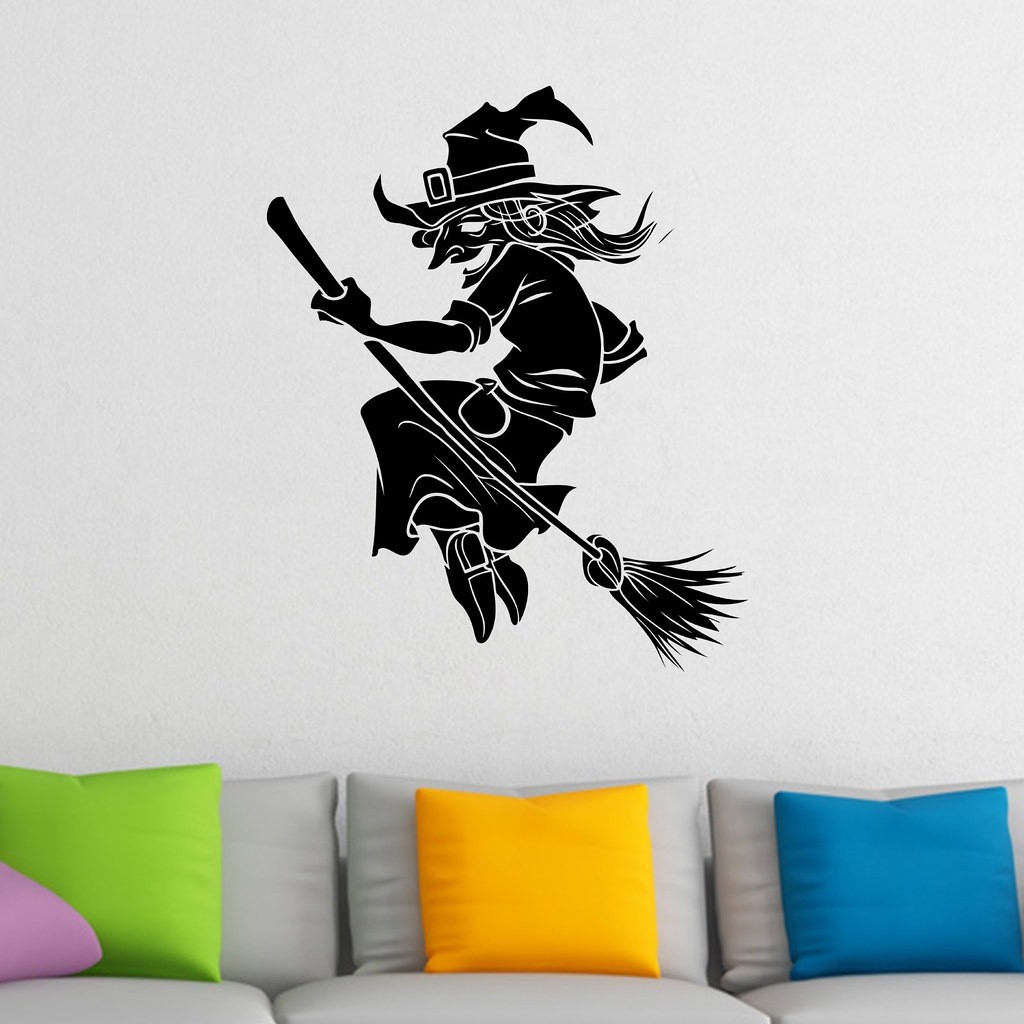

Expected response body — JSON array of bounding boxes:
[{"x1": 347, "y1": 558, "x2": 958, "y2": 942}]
[
  {"x1": 414, "y1": 784, "x2": 662, "y2": 980},
  {"x1": 0, "y1": 758, "x2": 221, "y2": 982},
  {"x1": 775, "y1": 786, "x2": 1023, "y2": 980},
  {"x1": 1, "y1": 861, "x2": 104, "y2": 984}
]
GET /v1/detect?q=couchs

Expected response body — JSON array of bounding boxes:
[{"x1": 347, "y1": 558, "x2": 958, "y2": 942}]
[{"x1": 0, "y1": 773, "x2": 1024, "y2": 1024}]
